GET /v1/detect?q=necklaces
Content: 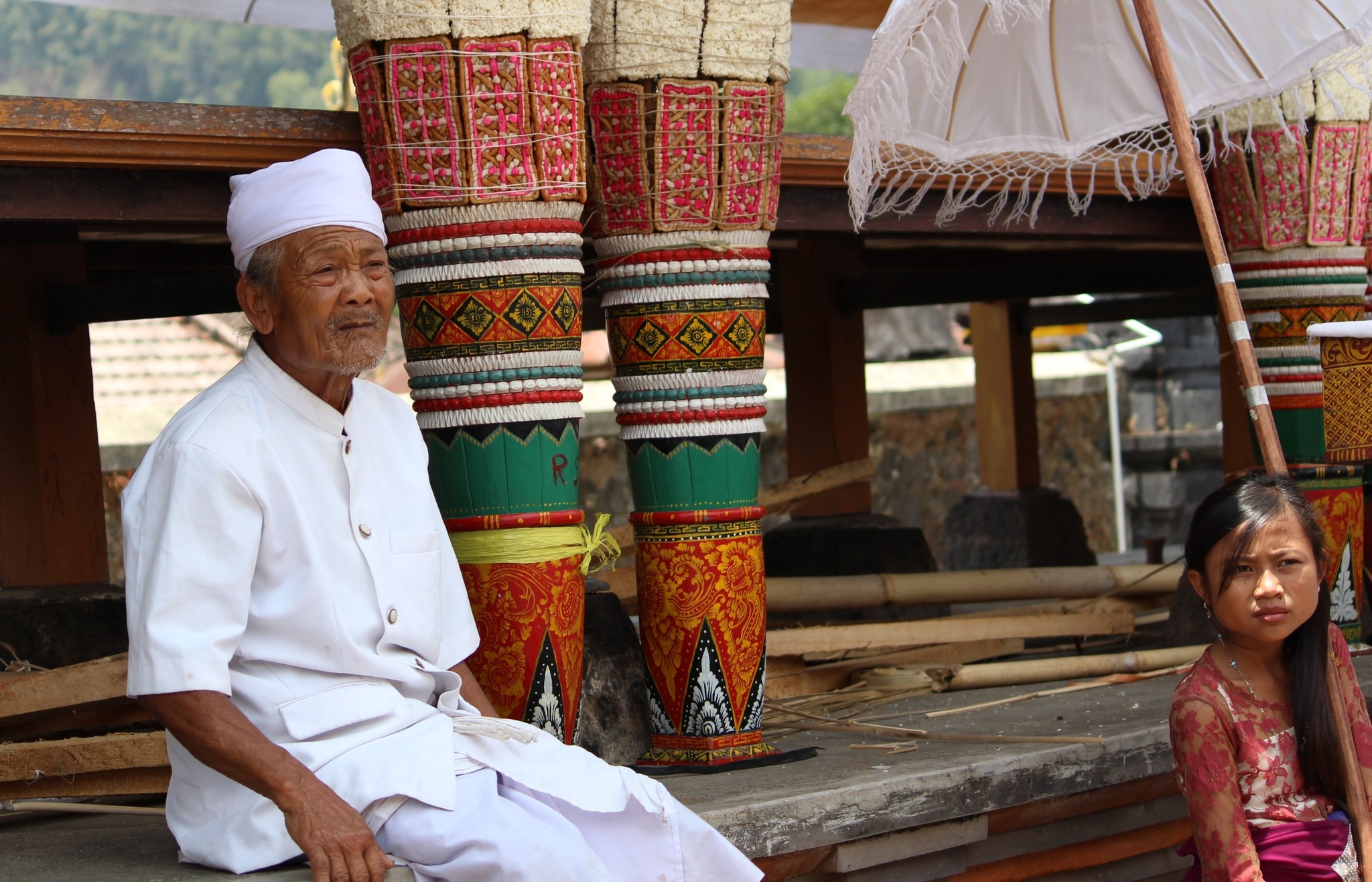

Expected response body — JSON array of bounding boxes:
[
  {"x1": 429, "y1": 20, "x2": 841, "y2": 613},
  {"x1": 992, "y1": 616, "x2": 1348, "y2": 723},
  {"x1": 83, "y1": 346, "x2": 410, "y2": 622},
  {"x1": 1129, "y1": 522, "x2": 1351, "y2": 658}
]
[{"x1": 1217, "y1": 631, "x2": 1296, "y2": 763}]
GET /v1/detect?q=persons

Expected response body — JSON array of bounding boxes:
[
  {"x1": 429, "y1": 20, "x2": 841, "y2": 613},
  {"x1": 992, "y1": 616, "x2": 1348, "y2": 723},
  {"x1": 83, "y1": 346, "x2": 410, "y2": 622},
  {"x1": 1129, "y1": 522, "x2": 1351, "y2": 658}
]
[
  {"x1": 1167, "y1": 474, "x2": 1372, "y2": 882},
  {"x1": 119, "y1": 148, "x2": 767, "y2": 882}
]
